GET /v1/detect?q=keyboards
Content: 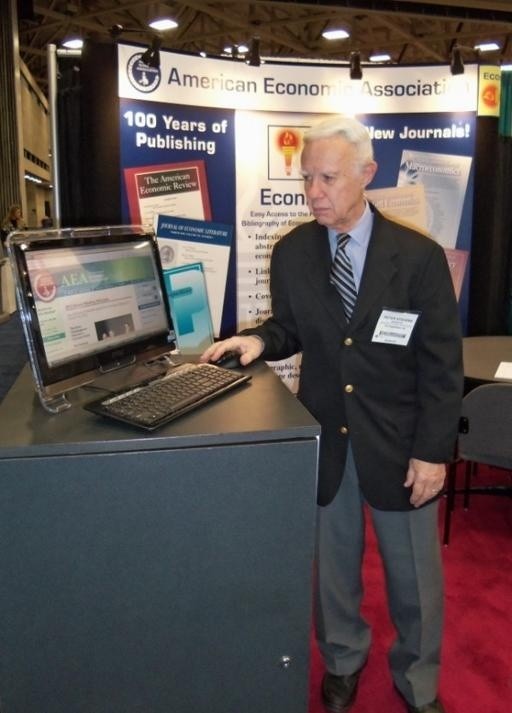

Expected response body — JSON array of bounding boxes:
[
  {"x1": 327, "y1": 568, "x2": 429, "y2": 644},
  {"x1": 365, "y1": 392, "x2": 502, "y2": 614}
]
[{"x1": 82, "y1": 362, "x2": 252, "y2": 433}]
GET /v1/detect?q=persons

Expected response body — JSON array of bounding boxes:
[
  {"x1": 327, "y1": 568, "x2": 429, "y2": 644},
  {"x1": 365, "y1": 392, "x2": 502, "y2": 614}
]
[
  {"x1": 1, "y1": 204, "x2": 26, "y2": 234},
  {"x1": 200, "y1": 115, "x2": 464, "y2": 712}
]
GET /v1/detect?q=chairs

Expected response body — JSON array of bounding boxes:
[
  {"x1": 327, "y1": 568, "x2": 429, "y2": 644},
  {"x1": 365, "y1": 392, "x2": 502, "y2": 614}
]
[{"x1": 442, "y1": 380, "x2": 512, "y2": 546}]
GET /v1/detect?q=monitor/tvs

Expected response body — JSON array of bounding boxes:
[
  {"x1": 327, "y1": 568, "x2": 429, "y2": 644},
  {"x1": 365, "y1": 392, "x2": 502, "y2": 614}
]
[{"x1": 5, "y1": 225, "x2": 183, "y2": 414}]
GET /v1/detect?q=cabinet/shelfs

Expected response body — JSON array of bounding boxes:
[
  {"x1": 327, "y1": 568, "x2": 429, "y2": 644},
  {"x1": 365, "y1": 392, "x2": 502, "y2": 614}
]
[{"x1": 0, "y1": 354, "x2": 322, "y2": 713}]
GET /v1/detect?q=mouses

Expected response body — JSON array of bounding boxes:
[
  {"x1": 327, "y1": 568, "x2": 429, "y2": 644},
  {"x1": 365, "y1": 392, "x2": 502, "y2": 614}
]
[{"x1": 208, "y1": 351, "x2": 244, "y2": 369}]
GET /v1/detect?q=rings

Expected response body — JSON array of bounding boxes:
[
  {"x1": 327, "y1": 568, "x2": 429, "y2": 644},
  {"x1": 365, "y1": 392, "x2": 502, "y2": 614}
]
[{"x1": 432, "y1": 489, "x2": 439, "y2": 495}]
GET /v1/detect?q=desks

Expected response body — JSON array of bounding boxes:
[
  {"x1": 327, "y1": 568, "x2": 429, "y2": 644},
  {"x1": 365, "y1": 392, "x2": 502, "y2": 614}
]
[{"x1": 463, "y1": 334, "x2": 512, "y2": 477}]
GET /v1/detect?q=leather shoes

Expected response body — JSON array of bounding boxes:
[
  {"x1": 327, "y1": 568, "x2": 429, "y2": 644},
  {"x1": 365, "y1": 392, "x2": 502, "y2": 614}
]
[
  {"x1": 394, "y1": 680, "x2": 444, "y2": 713},
  {"x1": 322, "y1": 654, "x2": 367, "y2": 712}
]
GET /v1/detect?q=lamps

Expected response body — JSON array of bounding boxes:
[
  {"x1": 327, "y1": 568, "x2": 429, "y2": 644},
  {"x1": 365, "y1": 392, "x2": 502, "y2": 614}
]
[
  {"x1": 448, "y1": 40, "x2": 466, "y2": 75},
  {"x1": 106, "y1": 22, "x2": 162, "y2": 71},
  {"x1": 231, "y1": 34, "x2": 262, "y2": 67},
  {"x1": 349, "y1": 48, "x2": 363, "y2": 81}
]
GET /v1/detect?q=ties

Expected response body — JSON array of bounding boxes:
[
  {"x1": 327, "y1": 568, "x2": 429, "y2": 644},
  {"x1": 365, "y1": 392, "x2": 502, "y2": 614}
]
[{"x1": 330, "y1": 232, "x2": 357, "y2": 325}]
